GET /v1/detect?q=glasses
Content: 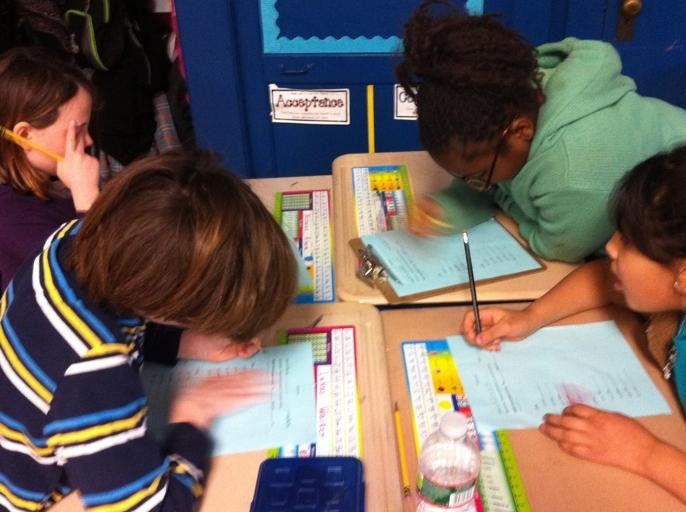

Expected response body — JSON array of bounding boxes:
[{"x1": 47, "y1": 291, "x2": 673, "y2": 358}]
[{"x1": 465, "y1": 124, "x2": 510, "y2": 193}]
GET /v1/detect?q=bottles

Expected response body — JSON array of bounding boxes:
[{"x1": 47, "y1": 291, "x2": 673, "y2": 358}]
[{"x1": 414, "y1": 413, "x2": 484, "y2": 506}]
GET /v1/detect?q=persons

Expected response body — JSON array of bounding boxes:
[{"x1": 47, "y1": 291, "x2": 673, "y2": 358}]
[
  {"x1": 0, "y1": 144, "x2": 299, "y2": 512},
  {"x1": 458, "y1": 140, "x2": 685, "y2": 507},
  {"x1": 0, "y1": 1, "x2": 200, "y2": 201},
  {"x1": 1, "y1": 48, "x2": 108, "y2": 299},
  {"x1": 391, "y1": 2, "x2": 686, "y2": 267}
]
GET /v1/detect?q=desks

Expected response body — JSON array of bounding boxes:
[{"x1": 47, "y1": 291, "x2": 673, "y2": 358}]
[
  {"x1": 330, "y1": 152, "x2": 589, "y2": 303},
  {"x1": 179, "y1": 303, "x2": 399, "y2": 511},
  {"x1": 382, "y1": 303, "x2": 685, "y2": 510},
  {"x1": 241, "y1": 174, "x2": 336, "y2": 299}
]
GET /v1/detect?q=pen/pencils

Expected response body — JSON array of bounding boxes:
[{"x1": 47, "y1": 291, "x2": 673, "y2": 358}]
[
  {"x1": 395, "y1": 402, "x2": 412, "y2": 502},
  {"x1": 463, "y1": 229, "x2": 481, "y2": 334},
  {"x1": 1, "y1": 126, "x2": 62, "y2": 160}
]
